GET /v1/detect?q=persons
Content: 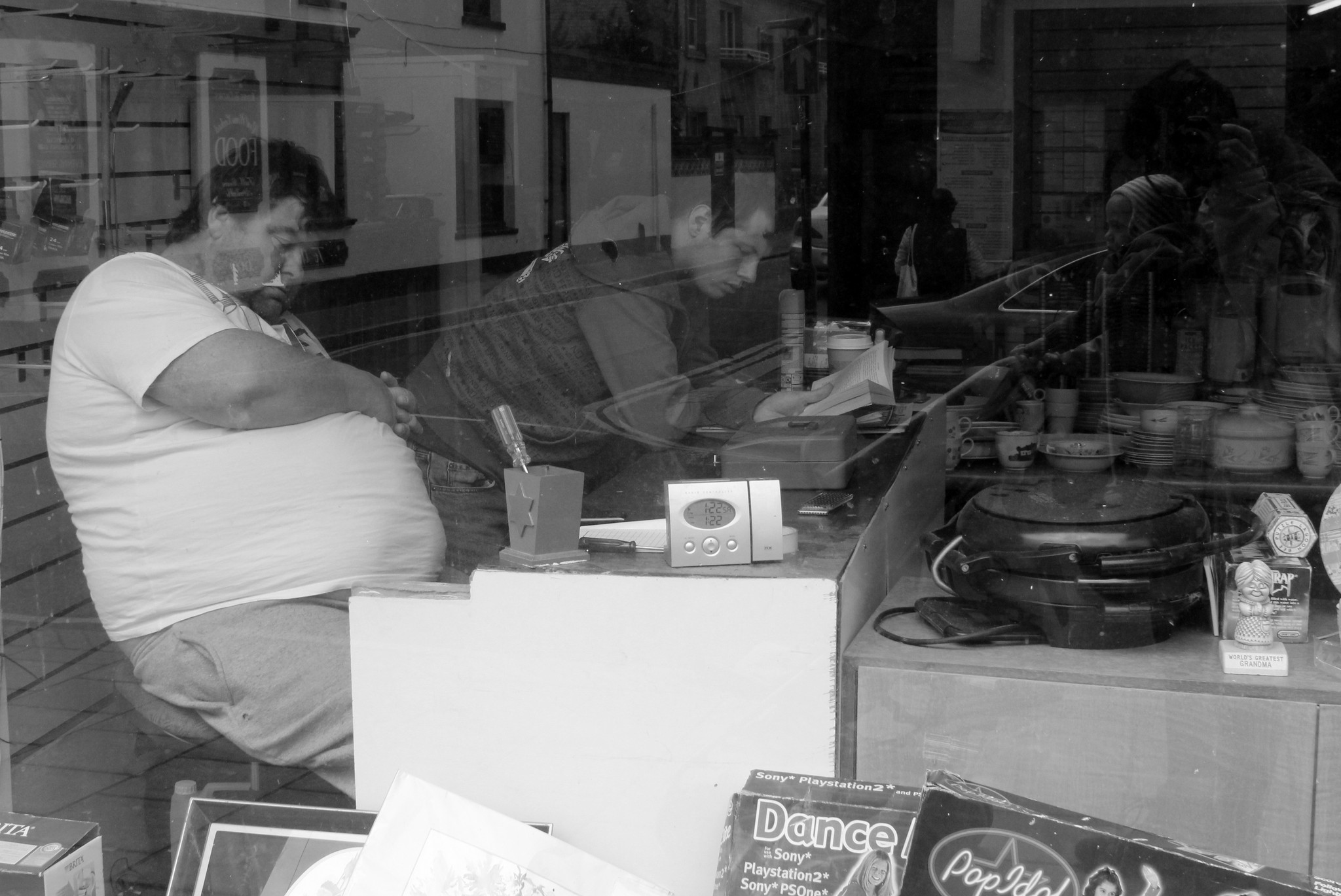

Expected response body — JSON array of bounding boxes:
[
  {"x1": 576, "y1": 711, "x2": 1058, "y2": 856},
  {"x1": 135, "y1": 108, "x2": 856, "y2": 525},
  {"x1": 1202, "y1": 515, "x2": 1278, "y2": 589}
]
[
  {"x1": 978, "y1": 296, "x2": 1135, "y2": 380},
  {"x1": 1122, "y1": 59, "x2": 1341, "y2": 298},
  {"x1": 896, "y1": 189, "x2": 986, "y2": 308},
  {"x1": 1088, "y1": 172, "x2": 1185, "y2": 370},
  {"x1": 398, "y1": 149, "x2": 836, "y2": 585}
]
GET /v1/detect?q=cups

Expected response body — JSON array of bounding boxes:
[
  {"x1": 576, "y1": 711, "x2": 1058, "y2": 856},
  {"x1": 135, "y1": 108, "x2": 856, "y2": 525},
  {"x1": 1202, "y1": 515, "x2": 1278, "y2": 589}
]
[{"x1": 827, "y1": 333, "x2": 873, "y2": 376}]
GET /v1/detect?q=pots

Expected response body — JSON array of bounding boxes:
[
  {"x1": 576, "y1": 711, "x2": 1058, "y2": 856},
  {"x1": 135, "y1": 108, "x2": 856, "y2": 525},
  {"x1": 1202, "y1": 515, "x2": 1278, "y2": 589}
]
[{"x1": 917, "y1": 476, "x2": 1264, "y2": 650}]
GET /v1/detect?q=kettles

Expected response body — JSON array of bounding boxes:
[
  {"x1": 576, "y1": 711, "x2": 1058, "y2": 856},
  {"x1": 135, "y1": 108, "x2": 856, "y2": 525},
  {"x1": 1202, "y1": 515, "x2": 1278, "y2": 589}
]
[{"x1": 1179, "y1": 272, "x2": 1337, "y2": 388}]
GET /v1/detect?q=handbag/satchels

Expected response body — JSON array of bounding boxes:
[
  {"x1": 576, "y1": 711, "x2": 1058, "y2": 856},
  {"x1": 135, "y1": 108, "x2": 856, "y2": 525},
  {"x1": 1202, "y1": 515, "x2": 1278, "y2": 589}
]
[{"x1": 897, "y1": 264, "x2": 920, "y2": 298}]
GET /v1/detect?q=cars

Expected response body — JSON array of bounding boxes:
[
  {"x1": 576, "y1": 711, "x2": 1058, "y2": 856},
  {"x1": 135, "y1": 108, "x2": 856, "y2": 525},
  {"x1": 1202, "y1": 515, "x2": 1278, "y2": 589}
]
[{"x1": 869, "y1": 236, "x2": 1112, "y2": 392}]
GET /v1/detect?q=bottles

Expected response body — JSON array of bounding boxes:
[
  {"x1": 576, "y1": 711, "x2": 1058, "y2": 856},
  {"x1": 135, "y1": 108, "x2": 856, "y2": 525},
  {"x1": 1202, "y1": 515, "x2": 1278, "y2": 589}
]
[{"x1": 776, "y1": 289, "x2": 806, "y2": 390}]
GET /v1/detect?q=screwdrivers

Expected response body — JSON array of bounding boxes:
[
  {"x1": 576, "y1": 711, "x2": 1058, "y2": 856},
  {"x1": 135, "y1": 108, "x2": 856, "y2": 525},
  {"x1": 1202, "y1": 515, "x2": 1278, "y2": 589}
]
[
  {"x1": 489, "y1": 404, "x2": 533, "y2": 475},
  {"x1": 576, "y1": 536, "x2": 664, "y2": 555}
]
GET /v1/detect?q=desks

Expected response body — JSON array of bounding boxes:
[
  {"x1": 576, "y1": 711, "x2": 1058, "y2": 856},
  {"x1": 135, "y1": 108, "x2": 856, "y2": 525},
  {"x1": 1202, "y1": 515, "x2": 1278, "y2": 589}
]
[{"x1": 345, "y1": 378, "x2": 942, "y2": 878}]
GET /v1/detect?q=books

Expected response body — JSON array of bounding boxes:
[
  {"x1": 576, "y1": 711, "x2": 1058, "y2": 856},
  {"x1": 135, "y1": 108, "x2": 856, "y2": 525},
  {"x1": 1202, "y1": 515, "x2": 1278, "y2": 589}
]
[{"x1": 799, "y1": 338, "x2": 897, "y2": 418}]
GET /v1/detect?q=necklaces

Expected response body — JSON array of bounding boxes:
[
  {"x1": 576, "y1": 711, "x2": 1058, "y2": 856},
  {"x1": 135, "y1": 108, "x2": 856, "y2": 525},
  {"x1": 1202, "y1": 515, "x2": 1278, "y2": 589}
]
[{"x1": 43, "y1": 135, "x2": 450, "y2": 808}]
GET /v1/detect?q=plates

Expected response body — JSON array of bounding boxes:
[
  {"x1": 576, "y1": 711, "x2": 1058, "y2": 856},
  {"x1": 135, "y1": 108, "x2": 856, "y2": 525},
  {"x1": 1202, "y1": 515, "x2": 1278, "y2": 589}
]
[{"x1": 947, "y1": 364, "x2": 1340, "y2": 484}]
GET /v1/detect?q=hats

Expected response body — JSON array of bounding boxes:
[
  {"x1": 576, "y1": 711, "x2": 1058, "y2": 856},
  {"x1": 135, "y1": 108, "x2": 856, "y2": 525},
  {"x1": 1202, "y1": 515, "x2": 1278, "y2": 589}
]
[{"x1": 1108, "y1": 174, "x2": 1188, "y2": 250}]
